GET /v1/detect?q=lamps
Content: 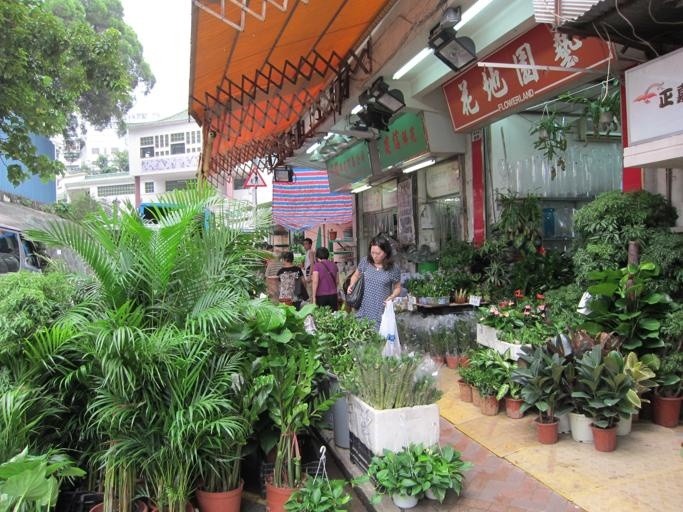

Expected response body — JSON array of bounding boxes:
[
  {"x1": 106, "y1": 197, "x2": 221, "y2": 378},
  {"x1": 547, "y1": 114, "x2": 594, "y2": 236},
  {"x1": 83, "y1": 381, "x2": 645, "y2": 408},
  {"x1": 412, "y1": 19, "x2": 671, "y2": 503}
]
[
  {"x1": 273, "y1": 167, "x2": 294, "y2": 182},
  {"x1": 349, "y1": 184, "x2": 372, "y2": 194},
  {"x1": 307, "y1": 0, "x2": 493, "y2": 194},
  {"x1": 402, "y1": 159, "x2": 436, "y2": 174}
]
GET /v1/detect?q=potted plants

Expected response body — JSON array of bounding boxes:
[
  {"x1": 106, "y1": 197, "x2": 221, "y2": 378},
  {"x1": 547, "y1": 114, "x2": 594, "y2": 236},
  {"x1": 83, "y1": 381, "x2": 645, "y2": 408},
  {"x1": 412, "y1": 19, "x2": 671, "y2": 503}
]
[
  {"x1": 557, "y1": 76, "x2": 621, "y2": 139},
  {"x1": 527, "y1": 110, "x2": 577, "y2": 162}
]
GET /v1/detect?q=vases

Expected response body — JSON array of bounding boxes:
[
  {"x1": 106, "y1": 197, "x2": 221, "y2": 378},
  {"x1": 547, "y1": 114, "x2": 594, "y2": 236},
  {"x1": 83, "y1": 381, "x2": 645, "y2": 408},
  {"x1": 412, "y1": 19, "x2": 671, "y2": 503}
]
[{"x1": 329, "y1": 226, "x2": 353, "y2": 241}]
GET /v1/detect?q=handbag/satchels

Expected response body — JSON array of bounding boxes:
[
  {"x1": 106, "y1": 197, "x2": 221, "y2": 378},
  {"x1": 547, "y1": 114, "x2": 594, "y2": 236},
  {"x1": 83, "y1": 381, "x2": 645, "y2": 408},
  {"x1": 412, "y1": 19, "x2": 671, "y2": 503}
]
[
  {"x1": 343, "y1": 271, "x2": 364, "y2": 309},
  {"x1": 295, "y1": 278, "x2": 309, "y2": 301},
  {"x1": 337, "y1": 288, "x2": 345, "y2": 302}
]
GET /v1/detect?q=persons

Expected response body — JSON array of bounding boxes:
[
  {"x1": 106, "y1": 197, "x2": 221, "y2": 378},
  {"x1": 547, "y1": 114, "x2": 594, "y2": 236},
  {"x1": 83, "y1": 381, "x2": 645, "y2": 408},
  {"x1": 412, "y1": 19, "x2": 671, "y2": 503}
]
[
  {"x1": 346, "y1": 235, "x2": 401, "y2": 333},
  {"x1": 312, "y1": 246, "x2": 339, "y2": 313},
  {"x1": 276, "y1": 251, "x2": 307, "y2": 312},
  {"x1": 302, "y1": 237, "x2": 315, "y2": 280},
  {"x1": 265, "y1": 245, "x2": 274, "y2": 268}
]
